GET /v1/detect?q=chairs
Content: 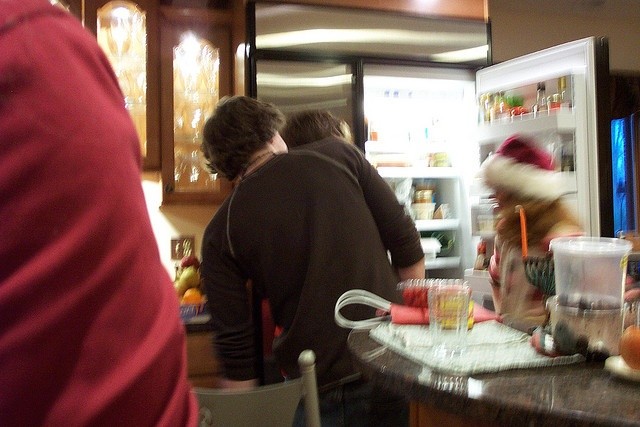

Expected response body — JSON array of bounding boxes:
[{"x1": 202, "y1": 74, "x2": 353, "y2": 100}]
[{"x1": 188, "y1": 350, "x2": 322, "y2": 427}]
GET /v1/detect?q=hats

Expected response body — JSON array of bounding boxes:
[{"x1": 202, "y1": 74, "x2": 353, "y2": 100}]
[{"x1": 478, "y1": 131, "x2": 563, "y2": 202}]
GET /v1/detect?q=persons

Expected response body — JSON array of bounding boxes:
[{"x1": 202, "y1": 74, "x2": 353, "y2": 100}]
[
  {"x1": 203, "y1": 95, "x2": 426, "y2": 427},
  {"x1": 278, "y1": 109, "x2": 353, "y2": 147},
  {"x1": 480, "y1": 136, "x2": 586, "y2": 332},
  {"x1": 0, "y1": 1, "x2": 200, "y2": 427}
]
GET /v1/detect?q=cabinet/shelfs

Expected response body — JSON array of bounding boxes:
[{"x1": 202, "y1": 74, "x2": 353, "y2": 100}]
[
  {"x1": 160, "y1": 19, "x2": 231, "y2": 207},
  {"x1": 68, "y1": 0, "x2": 159, "y2": 174}
]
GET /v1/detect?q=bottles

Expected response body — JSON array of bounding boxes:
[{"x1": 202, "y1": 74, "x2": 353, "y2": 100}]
[
  {"x1": 370, "y1": 120, "x2": 377, "y2": 141},
  {"x1": 480, "y1": 91, "x2": 511, "y2": 126},
  {"x1": 415, "y1": 190, "x2": 432, "y2": 203},
  {"x1": 558, "y1": 133, "x2": 576, "y2": 172},
  {"x1": 473, "y1": 242, "x2": 489, "y2": 270},
  {"x1": 531, "y1": 81, "x2": 548, "y2": 118},
  {"x1": 364, "y1": 115, "x2": 368, "y2": 142}
]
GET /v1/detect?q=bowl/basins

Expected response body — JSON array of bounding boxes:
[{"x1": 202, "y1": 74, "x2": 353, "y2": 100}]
[
  {"x1": 557, "y1": 77, "x2": 573, "y2": 114},
  {"x1": 549, "y1": 236, "x2": 633, "y2": 310},
  {"x1": 478, "y1": 214, "x2": 494, "y2": 232},
  {"x1": 414, "y1": 179, "x2": 436, "y2": 192},
  {"x1": 546, "y1": 295, "x2": 630, "y2": 363}
]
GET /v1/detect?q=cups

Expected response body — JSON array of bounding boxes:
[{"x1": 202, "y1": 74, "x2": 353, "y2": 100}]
[{"x1": 427, "y1": 287, "x2": 469, "y2": 359}]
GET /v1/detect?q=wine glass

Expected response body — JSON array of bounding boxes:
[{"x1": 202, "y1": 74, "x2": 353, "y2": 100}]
[
  {"x1": 97, "y1": 0, "x2": 147, "y2": 157},
  {"x1": 173, "y1": 28, "x2": 220, "y2": 193}
]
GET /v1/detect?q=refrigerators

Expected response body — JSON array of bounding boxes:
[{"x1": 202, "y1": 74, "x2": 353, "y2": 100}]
[{"x1": 229, "y1": 0, "x2": 614, "y2": 309}]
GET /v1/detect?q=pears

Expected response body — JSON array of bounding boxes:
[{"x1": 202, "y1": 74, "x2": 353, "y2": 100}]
[
  {"x1": 172, "y1": 278, "x2": 187, "y2": 296},
  {"x1": 181, "y1": 267, "x2": 199, "y2": 288}
]
